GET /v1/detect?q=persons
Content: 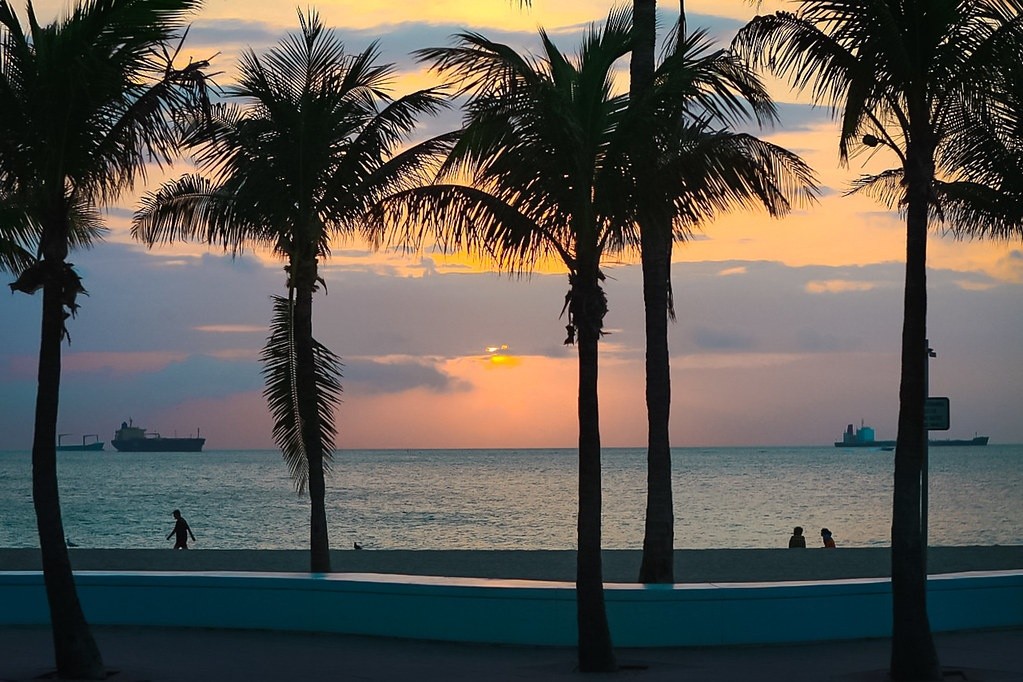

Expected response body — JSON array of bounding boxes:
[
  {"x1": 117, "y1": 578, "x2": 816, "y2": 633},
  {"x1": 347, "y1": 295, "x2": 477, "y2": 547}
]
[
  {"x1": 789, "y1": 527, "x2": 807, "y2": 548},
  {"x1": 166, "y1": 509, "x2": 196, "y2": 550},
  {"x1": 820, "y1": 529, "x2": 836, "y2": 548}
]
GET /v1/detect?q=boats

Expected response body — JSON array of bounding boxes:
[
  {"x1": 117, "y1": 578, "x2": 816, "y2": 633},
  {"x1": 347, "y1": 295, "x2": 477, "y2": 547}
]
[
  {"x1": 111, "y1": 418, "x2": 205, "y2": 453},
  {"x1": 55, "y1": 432, "x2": 104, "y2": 451},
  {"x1": 834, "y1": 418, "x2": 989, "y2": 447}
]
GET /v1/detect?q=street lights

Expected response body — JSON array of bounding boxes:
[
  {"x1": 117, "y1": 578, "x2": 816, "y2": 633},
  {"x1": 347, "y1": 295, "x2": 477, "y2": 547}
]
[{"x1": 920, "y1": 336, "x2": 936, "y2": 589}]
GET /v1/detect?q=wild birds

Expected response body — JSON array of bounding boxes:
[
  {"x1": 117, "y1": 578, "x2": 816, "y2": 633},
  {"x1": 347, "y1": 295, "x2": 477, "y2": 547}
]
[
  {"x1": 354, "y1": 542, "x2": 362, "y2": 550},
  {"x1": 66, "y1": 538, "x2": 79, "y2": 548}
]
